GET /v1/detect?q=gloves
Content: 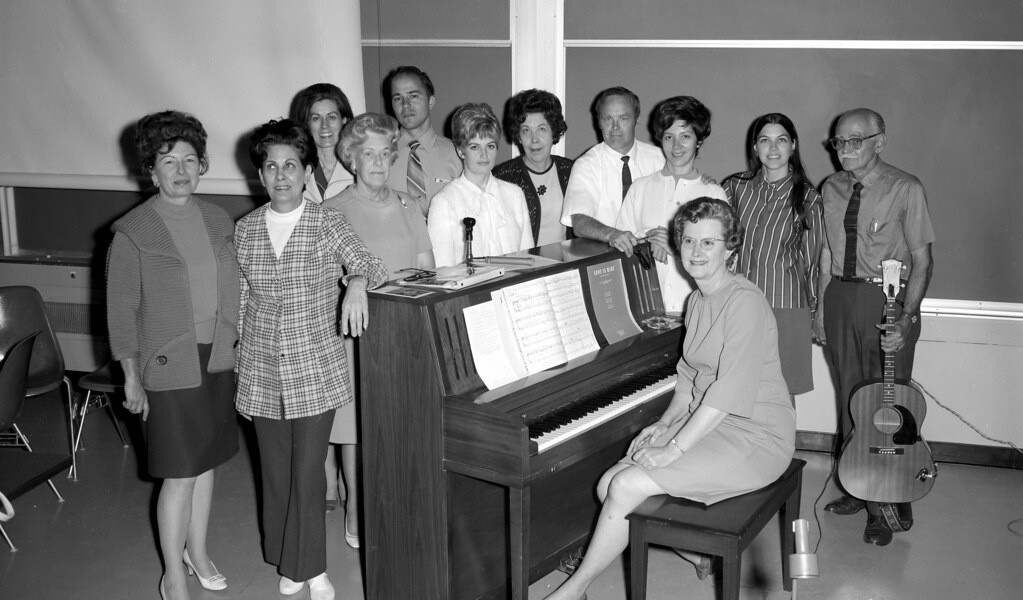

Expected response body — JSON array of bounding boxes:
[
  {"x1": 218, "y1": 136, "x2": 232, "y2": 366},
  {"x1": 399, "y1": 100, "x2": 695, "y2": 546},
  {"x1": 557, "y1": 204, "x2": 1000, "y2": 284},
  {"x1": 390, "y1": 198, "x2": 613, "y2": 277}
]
[{"x1": 632, "y1": 233, "x2": 657, "y2": 270}]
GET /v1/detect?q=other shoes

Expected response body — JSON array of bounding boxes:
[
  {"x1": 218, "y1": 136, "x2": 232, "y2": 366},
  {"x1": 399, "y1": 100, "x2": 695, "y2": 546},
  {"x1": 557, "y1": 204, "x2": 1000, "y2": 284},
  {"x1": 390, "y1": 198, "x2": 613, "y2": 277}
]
[
  {"x1": 278, "y1": 575, "x2": 305, "y2": 595},
  {"x1": 697, "y1": 551, "x2": 715, "y2": 581},
  {"x1": 306, "y1": 572, "x2": 336, "y2": 600},
  {"x1": 326, "y1": 496, "x2": 348, "y2": 512},
  {"x1": 345, "y1": 511, "x2": 364, "y2": 548}
]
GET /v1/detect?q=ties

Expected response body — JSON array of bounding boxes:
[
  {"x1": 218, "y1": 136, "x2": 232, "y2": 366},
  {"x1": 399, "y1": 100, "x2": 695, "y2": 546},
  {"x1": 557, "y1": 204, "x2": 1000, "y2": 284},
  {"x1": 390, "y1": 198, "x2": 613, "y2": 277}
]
[
  {"x1": 843, "y1": 182, "x2": 864, "y2": 281},
  {"x1": 406, "y1": 140, "x2": 428, "y2": 219},
  {"x1": 620, "y1": 155, "x2": 633, "y2": 203}
]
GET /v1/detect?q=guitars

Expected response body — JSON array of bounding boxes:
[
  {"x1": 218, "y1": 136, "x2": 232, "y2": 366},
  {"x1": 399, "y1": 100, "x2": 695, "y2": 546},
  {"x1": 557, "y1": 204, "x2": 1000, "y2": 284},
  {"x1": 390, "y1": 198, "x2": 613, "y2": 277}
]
[{"x1": 834, "y1": 257, "x2": 940, "y2": 503}]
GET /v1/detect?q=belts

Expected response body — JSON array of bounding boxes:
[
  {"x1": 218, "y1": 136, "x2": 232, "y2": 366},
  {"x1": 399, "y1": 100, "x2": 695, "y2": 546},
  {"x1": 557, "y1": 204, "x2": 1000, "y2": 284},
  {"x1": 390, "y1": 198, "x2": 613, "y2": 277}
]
[{"x1": 836, "y1": 275, "x2": 908, "y2": 286}]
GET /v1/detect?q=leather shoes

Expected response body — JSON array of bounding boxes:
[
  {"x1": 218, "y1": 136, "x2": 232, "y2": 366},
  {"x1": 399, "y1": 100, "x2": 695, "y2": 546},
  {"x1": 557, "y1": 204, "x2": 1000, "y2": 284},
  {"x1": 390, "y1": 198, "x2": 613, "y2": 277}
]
[
  {"x1": 824, "y1": 493, "x2": 868, "y2": 515},
  {"x1": 864, "y1": 512, "x2": 894, "y2": 546}
]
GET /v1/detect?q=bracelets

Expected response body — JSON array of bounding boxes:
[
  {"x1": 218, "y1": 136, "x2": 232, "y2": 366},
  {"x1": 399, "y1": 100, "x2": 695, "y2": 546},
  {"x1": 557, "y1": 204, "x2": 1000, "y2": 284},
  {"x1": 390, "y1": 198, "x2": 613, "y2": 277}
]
[{"x1": 672, "y1": 439, "x2": 685, "y2": 453}]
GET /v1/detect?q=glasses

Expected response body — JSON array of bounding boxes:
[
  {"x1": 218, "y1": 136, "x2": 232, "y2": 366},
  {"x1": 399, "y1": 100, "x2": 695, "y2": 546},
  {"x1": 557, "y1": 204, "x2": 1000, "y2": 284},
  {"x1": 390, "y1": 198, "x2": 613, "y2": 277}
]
[
  {"x1": 400, "y1": 266, "x2": 438, "y2": 282},
  {"x1": 830, "y1": 131, "x2": 883, "y2": 150},
  {"x1": 681, "y1": 235, "x2": 727, "y2": 251}
]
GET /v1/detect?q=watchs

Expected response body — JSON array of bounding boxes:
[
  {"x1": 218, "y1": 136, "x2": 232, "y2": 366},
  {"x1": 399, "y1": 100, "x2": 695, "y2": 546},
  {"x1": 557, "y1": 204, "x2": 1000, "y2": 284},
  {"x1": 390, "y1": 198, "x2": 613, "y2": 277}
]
[{"x1": 903, "y1": 312, "x2": 918, "y2": 324}]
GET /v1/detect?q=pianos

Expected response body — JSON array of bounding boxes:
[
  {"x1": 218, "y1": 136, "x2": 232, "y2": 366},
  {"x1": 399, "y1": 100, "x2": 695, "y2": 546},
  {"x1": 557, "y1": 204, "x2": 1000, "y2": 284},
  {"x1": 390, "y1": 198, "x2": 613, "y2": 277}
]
[{"x1": 356, "y1": 239, "x2": 685, "y2": 600}]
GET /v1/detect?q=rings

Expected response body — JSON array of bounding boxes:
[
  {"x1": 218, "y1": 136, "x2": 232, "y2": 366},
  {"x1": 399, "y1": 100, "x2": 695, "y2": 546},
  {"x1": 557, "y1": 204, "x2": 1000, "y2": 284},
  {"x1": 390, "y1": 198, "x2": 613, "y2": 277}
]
[{"x1": 896, "y1": 346, "x2": 899, "y2": 350}]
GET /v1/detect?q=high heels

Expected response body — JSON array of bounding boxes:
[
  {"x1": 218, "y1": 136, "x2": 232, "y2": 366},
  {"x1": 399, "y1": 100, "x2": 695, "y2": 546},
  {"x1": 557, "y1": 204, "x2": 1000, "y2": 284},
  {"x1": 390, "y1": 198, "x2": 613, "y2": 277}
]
[
  {"x1": 183, "y1": 549, "x2": 228, "y2": 591},
  {"x1": 160, "y1": 574, "x2": 167, "y2": 600}
]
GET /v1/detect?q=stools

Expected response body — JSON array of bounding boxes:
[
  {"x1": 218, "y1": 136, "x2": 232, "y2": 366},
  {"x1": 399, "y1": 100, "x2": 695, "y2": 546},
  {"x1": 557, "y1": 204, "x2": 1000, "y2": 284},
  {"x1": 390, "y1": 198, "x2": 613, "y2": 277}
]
[{"x1": 625, "y1": 457, "x2": 807, "y2": 600}]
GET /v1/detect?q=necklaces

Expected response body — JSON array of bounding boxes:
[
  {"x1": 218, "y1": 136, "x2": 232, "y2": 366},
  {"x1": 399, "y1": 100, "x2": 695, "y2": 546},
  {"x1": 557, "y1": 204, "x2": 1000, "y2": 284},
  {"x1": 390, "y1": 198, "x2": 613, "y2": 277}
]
[{"x1": 536, "y1": 184, "x2": 548, "y2": 195}]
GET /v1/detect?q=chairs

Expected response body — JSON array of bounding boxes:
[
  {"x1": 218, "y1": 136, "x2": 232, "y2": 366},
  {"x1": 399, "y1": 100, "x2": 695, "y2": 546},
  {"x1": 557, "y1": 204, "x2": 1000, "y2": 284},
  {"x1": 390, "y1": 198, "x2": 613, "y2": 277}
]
[
  {"x1": 0, "y1": 328, "x2": 72, "y2": 555},
  {"x1": 65, "y1": 355, "x2": 131, "y2": 480},
  {"x1": 0, "y1": 284, "x2": 79, "y2": 483}
]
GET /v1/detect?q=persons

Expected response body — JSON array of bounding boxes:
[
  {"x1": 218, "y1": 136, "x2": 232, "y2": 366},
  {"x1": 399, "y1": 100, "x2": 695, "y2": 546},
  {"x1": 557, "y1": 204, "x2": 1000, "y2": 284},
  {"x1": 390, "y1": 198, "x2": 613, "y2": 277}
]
[
  {"x1": 289, "y1": 83, "x2": 356, "y2": 203},
  {"x1": 232, "y1": 119, "x2": 389, "y2": 600},
  {"x1": 491, "y1": 89, "x2": 575, "y2": 249},
  {"x1": 722, "y1": 113, "x2": 825, "y2": 409},
  {"x1": 105, "y1": 112, "x2": 237, "y2": 600},
  {"x1": 425, "y1": 102, "x2": 535, "y2": 268},
  {"x1": 315, "y1": 112, "x2": 435, "y2": 550},
  {"x1": 384, "y1": 66, "x2": 464, "y2": 205},
  {"x1": 811, "y1": 108, "x2": 936, "y2": 545},
  {"x1": 617, "y1": 96, "x2": 739, "y2": 313},
  {"x1": 544, "y1": 197, "x2": 796, "y2": 600},
  {"x1": 559, "y1": 87, "x2": 718, "y2": 257}
]
